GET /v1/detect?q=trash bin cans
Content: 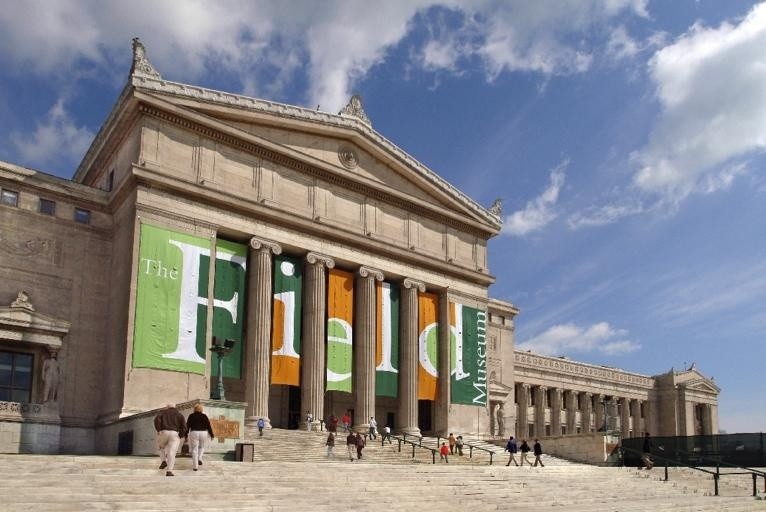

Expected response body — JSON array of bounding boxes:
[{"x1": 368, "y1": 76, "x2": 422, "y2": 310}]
[{"x1": 236, "y1": 443, "x2": 254, "y2": 462}]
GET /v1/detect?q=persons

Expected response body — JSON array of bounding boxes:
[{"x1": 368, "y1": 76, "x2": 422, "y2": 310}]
[
  {"x1": 519, "y1": 439, "x2": 534, "y2": 466},
  {"x1": 639, "y1": 431, "x2": 655, "y2": 470},
  {"x1": 257, "y1": 416, "x2": 264, "y2": 436},
  {"x1": 186, "y1": 403, "x2": 215, "y2": 471},
  {"x1": 533, "y1": 438, "x2": 545, "y2": 466},
  {"x1": 303, "y1": 408, "x2": 465, "y2": 463},
  {"x1": 504, "y1": 435, "x2": 519, "y2": 466},
  {"x1": 153, "y1": 402, "x2": 188, "y2": 476},
  {"x1": 599, "y1": 427, "x2": 604, "y2": 432}
]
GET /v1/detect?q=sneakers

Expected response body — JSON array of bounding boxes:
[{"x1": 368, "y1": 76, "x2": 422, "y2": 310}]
[
  {"x1": 166, "y1": 472, "x2": 174, "y2": 476},
  {"x1": 159, "y1": 461, "x2": 167, "y2": 469}
]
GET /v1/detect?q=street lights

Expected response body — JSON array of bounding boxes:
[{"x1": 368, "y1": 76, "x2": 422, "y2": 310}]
[
  {"x1": 209, "y1": 335, "x2": 235, "y2": 400},
  {"x1": 598, "y1": 394, "x2": 621, "y2": 431}
]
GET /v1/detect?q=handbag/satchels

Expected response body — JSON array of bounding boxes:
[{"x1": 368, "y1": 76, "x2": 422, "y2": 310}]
[{"x1": 181, "y1": 444, "x2": 189, "y2": 454}]
